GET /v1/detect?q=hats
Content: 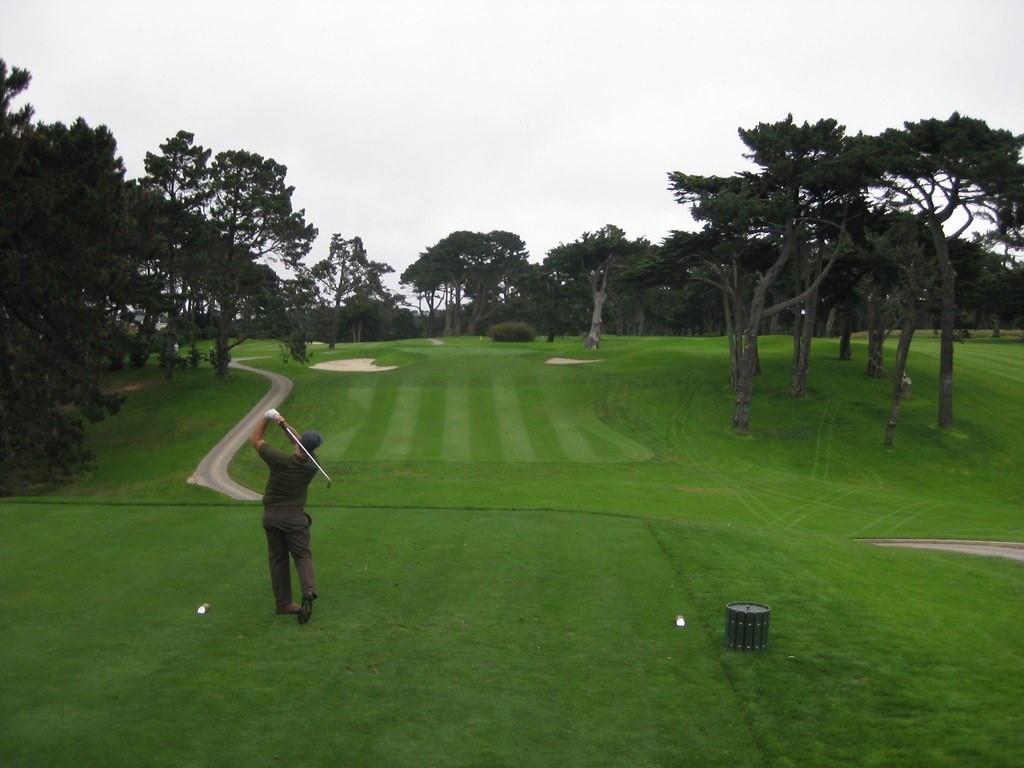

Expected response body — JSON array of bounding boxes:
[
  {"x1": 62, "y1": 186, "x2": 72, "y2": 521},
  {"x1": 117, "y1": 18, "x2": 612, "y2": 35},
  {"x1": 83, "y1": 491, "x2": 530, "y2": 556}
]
[{"x1": 299, "y1": 431, "x2": 322, "y2": 450}]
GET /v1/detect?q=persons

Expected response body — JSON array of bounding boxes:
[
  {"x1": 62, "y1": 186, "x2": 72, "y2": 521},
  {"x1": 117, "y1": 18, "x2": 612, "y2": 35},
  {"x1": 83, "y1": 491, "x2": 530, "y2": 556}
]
[{"x1": 250, "y1": 409, "x2": 322, "y2": 624}]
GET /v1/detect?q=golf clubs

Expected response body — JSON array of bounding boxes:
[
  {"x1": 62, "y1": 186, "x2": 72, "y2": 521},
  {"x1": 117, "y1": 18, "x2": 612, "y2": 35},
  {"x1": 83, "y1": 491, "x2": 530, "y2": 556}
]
[{"x1": 281, "y1": 421, "x2": 332, "y2": 489}]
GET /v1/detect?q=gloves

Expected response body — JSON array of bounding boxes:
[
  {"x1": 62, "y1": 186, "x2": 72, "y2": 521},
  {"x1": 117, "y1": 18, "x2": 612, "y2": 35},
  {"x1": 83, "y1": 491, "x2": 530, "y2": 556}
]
[{"x1": 264, "y1": 408, "x2": 279, "y2": 420}]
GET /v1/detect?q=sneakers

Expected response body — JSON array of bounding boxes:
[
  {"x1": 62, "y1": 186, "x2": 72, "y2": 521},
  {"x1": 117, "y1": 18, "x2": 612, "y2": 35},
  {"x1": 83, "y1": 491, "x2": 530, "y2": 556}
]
[
  {"x1": 275, "y1": 602, "x2": 301, "y2": 613},
  {"x1": 297, "y1": 590, "x2": 313, "y2": 624}
]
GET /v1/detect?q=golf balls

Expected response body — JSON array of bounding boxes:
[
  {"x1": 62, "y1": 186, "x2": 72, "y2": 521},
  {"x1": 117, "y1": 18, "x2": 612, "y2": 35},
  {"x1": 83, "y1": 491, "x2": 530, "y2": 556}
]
[
  {"x1": 677, "y1": 618, "x2": 685, "y2": 627},
  {"x1": 197, "y1": 607, "x2": 206, "y2": 614}
]
